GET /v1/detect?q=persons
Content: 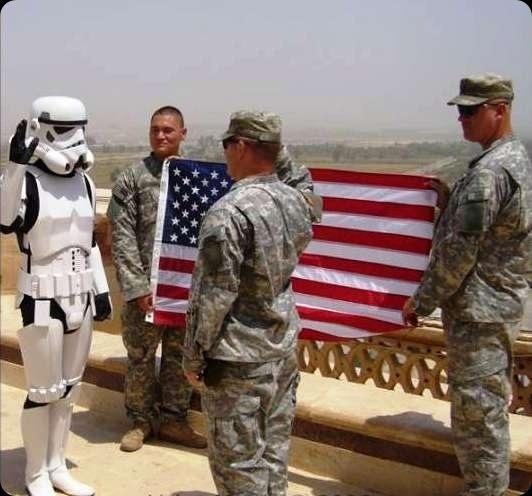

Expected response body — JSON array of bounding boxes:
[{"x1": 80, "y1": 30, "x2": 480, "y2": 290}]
[
  {"x1": 402, "y1": 73, "x2": 532, "y2": 496},
  {"x1": 182, "y1": 110, "x2": 323, "y2": 496},
  {"x1": 0, "y1": 95, "x2": 114, "y2": 496},
  {"x1": 106, "y1": 106, "x2": 207, "y2": 451}
]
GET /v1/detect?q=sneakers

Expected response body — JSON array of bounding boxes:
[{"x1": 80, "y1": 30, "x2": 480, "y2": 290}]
[
  {"x1": 158, "y1": 421, "x2": 207, "y2": 448},
  {"x1": 121, "y1": 420, "x2": 154, "y2": 451}
]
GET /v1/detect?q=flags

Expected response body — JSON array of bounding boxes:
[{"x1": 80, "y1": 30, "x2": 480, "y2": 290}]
[{"x1": 145, "y1": 158, "x2": 438, "y2": 342}]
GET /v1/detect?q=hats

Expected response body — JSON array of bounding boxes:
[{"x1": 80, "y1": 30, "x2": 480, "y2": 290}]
[
  {"x1": 447, "y1": 74, "x2": 514, "y2": 106},
  {"x1": 216, "y1": 109, "x2": 282, "y2": 144}
]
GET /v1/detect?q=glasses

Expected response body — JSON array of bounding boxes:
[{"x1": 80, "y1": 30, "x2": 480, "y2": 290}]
[{"x1": 458, "y1": 106, "x2": 485, "y2": 117}]
